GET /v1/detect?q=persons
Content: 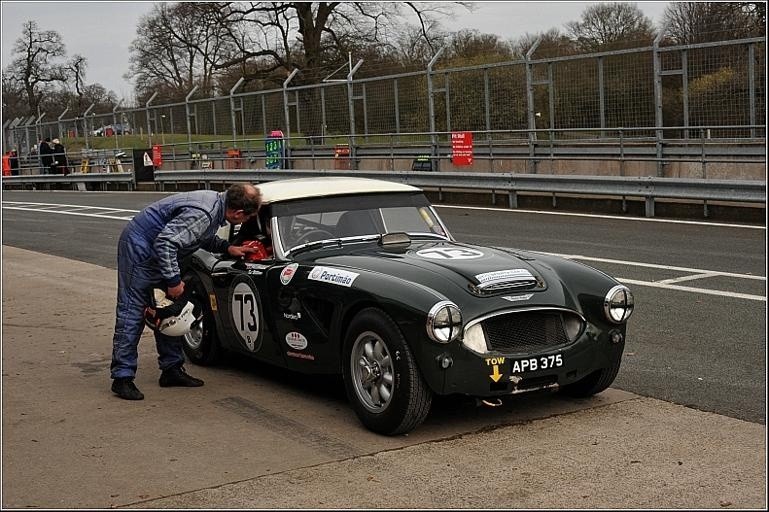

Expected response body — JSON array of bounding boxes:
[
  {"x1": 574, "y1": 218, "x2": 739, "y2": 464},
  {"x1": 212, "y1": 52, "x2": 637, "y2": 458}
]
[
  {"x1": 233, "y1": 212, "x2": 296, "y2": 261},
  {"x1": 2, "y1": 106, "x2": 94, "y2": 189},
  {"x1": 109, "y1": 181, "x2": 264, "y2": 400}
]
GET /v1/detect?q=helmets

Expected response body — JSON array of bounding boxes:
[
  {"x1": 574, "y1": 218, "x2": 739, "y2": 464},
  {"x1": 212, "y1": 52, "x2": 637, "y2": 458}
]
[{"x1": 142, "y1": 293, "x2": 203, "y2": 337}]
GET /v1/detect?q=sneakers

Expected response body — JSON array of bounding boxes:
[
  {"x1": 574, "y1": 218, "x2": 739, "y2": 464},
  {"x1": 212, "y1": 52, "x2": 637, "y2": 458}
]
[
  {"x1": 159, "y1": 364, "x2": 204, "y2": 387},
  {"x1": 111, "y1": 377, "x2": 144, "y2": 400}
]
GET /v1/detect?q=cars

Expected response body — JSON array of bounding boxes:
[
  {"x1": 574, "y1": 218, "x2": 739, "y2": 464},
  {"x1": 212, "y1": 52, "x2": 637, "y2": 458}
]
[
  {"x1": 175, "y1": 173, "x2": 638, "y2": 439},
  {"x1": 89, "y1": 123, "x2": 133, "y2": 137}
]
